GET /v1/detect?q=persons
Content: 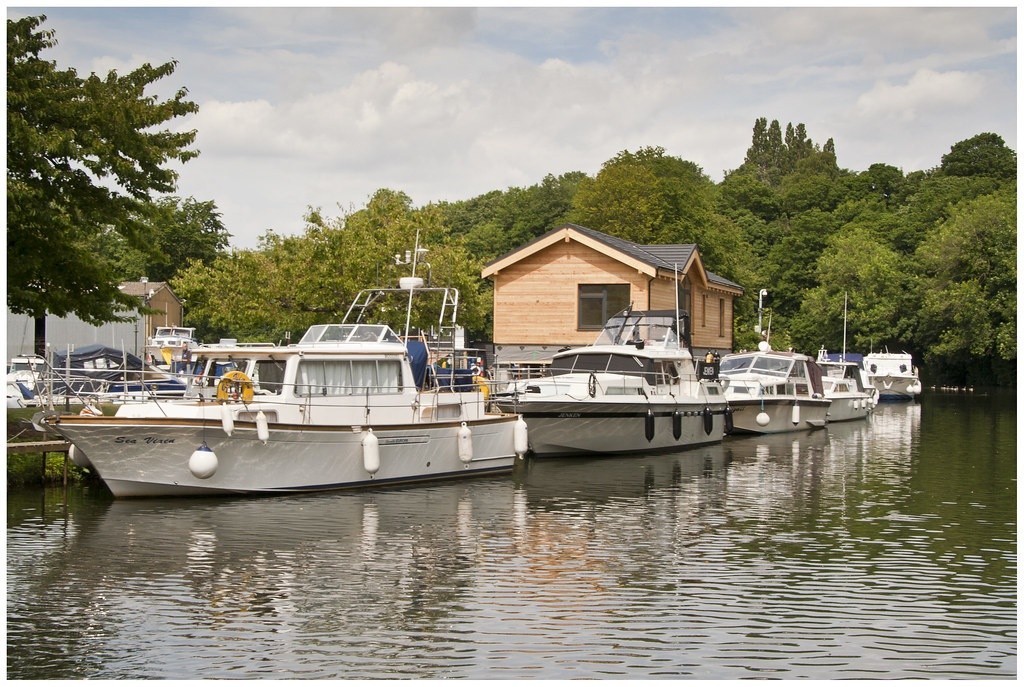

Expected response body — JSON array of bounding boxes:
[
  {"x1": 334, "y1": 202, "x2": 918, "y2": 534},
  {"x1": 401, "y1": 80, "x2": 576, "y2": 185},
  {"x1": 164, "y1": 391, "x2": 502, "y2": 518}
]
[
  {"x1": 181, "y1": 343, "x2": 192, "y2": 362},
  {"x1": 699, "y1": 349, "x2": 720, "y2": 382}
]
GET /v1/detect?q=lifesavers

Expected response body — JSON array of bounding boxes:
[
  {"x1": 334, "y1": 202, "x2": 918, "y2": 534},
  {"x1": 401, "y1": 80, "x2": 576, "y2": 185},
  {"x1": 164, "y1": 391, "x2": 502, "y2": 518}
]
[
  {"x1": 216, "y1": 370, "x2": 254, "y2": 405},
  {"x1": 470, "y1": 365, "x2": 481, "y2": 375},
  {"x1": 472, "y1": 375, "x2": 490, "y2": 405}
]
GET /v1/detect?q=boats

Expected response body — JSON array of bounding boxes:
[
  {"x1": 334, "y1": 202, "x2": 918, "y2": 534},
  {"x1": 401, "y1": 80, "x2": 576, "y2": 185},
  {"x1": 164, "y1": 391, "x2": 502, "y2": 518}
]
[{"x1": 9, "y1": 230, "x2": 922, "y2": 497}]
